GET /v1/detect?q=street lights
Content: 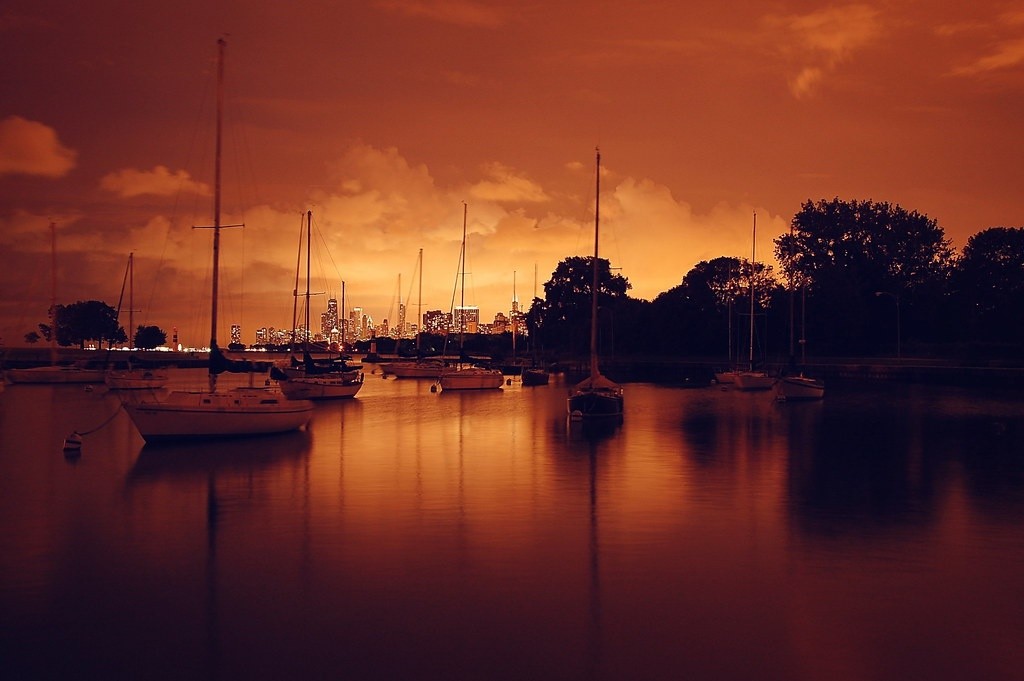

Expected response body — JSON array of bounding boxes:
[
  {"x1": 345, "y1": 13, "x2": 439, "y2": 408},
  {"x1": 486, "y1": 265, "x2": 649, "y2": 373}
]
[
  {"x1": 873, "y1": 291, "x2": 901, "y2": 364},
  {"x1": 598, "y1": 306, "x2": 616, "y2": 361}
]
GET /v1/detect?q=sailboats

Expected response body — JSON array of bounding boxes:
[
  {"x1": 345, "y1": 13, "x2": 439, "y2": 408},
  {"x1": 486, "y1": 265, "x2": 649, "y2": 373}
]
[
  {"x1": 103, "y1": 30, "x2": 315, "y2": 445},
  {"x1": 770, "y1": 224, "x2": 824, "y2": 399},
  {"x1": 563, "y1": 145, "x2": 627, "y2": 418},
  {"x1": 711, "y1": 263, "x2": 743, "y2": 384},
  {"x1": 103, "y1": 249, "x2": 170, "y2": 389},
  {"x1": 436, "y1": 202, "x2": 505, "y2": 390},
  {"x1": 268, "y1": 210, "x2": 365, "y2": 402},
  {"x1": 520, "y1": 264, "x2": 551, "y2": 387},
  {"x1": 378, "y1": 248, "x2": 450, "y2": 379},
  {"x1": 0, "y1": 218, "x2": 108, "y2": 386},
  {"x1": 732, "y1": 208, "x2": 776, "y2": 390}
]
